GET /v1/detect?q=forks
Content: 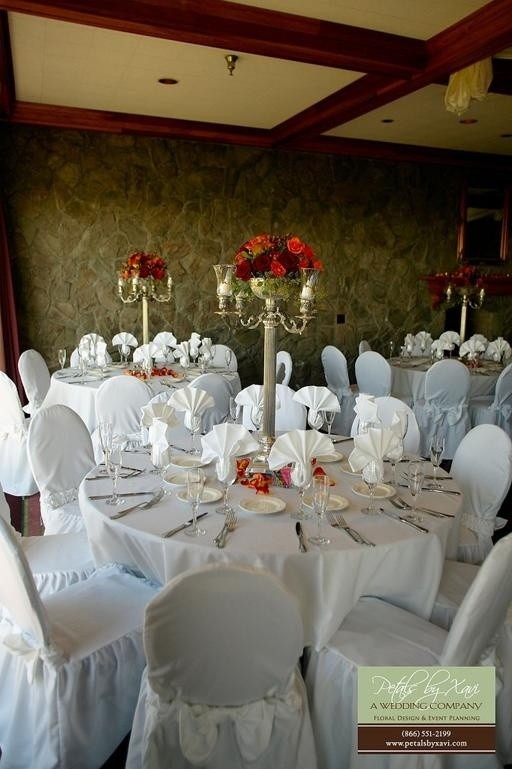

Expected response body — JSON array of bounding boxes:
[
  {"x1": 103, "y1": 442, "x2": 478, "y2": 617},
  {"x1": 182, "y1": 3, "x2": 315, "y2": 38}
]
[
  {"x1": 326, "y1": 510, "x2": 376, "y2": 549},
  {"x1": 389, "y1": 495, "x2": 456, "y2": 521},
  {"x1": 213, "y1": 511, "x2": 237, "y2": 549},
  {"x1": 403, "y1": 471, "x2": 454, "y2": 480},
  {"x1": 85, "y1": 469, "x2": 136, "y2": 483}
]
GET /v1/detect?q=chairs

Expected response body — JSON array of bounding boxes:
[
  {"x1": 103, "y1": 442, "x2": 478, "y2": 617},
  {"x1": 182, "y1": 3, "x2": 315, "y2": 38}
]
[
  {"x1": 430, "y1": 559, "x2": 512, "y2": 765},
  {"x1": 349, "y1": 396, "x2": 421, "y2": 455},
  {"x1": 302, "y1": 531, "x2": 512, "y2": 769},
  {"x1": 449, "y1": 422, "x2": 512, "y2": 566},
  {"x1": 0, "y1": 514, "x2": 162, "y2": 769},
  {"x1": 414, "y1": 358, "x2": 473, "y2": 460},
  {"x1": 198, "y1": 344, "x2": 238, "y2": 371},
  {"x1": 354, "y1": 350, "x2": 414, "y2": 409},
  {"x1": 124, "y1": 559, "x2": 318, "y2": 769},
  {"x1": 276, "y1": 350, "x2": 293, "y2": 386},
  {"x1": 358, "y1": 340, "x2": 372, "y2": 356},
  {"x1": 242, "y1": 383, "x2": 308, "y2": 430},
  {"x1": 468, "y1": 363, "x2": 512, "y2": 441},
  {"x1": 187, "y1": 373, "x2": 235, "y2": 424},
  {"x1": 70, "y1": 346, "x2": 113, "y2": 368},
  {"x1": 0, "y1": 370, "x2": 40, "y2": 497},
  {"x1": 27, "y1": 404, "x2": 97, "y2": 537},
  {"x1": 469, "y1": 333, "x2": 489, "y2": 348},
  {"x1": 321, "y1": 344, "x2": 357, "y2": 437},
  {"x1": 133, "y1": 343, "x2": 175, "y2": 363},
  {"x1": 1, "y1": 484, "x2": 96, "y2": 600},
  {"x1": 439, "y1": 331, "x2": 460, "y2": 347},
  {"x1": 95, "y1": 375, "x2": 155, "y2": 454},
  {"x1": 17, "y1": 348, "x2": 51, "y2": 418}
]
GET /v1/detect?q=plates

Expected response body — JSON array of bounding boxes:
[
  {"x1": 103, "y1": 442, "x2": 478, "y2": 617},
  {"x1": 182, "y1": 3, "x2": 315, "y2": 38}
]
[
  {"x1": 163, "y1": 454, "x2": 287, "y2": 514},
  {"x1": 86, "y1": 365, "x2": 126, "y2": 378},
  {"x1": 302, "y1": 451, "x2": 397, "y2": 511}
]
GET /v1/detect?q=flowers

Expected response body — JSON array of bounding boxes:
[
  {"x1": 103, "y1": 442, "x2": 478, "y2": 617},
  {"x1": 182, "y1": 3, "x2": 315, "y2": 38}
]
[
  {"x1": 227, "y1": 232, "x2": 329, "y2": 308},
  {"x1": 435, "y1": 266, "x2": 496, "y2": 301},
  {"x1": 119, "y1": 248, "x2": 171, "y2": 295}
]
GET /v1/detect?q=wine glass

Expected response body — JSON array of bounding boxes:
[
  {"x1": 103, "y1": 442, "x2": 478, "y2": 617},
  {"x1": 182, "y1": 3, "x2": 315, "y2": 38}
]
[
  {"x1": 58, "y1": 339, "x2": 231, "y2": 385},
  {"x1": 99, "y1": 397, "x2": 263, "y2": 537},
  {"x1": 388, "y1": 336, "x2": 512, "y2": 374},
  {"x1": 290, "y1": 406, "x2": 445, "y2": 545}
]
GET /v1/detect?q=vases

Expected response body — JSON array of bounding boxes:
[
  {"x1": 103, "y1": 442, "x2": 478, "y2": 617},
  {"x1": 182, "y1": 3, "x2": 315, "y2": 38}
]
[{"x1": 248, "y1": 277, "x2": 300, "y2": 308}]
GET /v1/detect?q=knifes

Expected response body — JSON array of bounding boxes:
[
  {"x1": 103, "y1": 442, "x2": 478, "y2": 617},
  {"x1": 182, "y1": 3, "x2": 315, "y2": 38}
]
[
  {"x1": 87, "y1": 491, "x2": 154, "y2": 501},
  {"x1": 398, "y1": 484, "x2": 461, "y2": 496},
  {"x1": 294, "y1": 519, "x2": 308, "y2": 555},
  {"x1": 160, "y1": 513, "x2": 207, "y2": 539}
]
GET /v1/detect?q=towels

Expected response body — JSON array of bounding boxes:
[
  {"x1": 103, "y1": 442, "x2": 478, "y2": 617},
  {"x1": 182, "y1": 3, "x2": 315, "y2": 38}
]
[
  {"x1": 187, "y1": 332, "x2": 202, "y2": 357},
  {"x1": 152, "y1": 332, "x2": 180, "y2": 353},
  {"x1": 385, "y1": 409, "x2": 408, "y2": 461},
  {"x1": 141, "y1": 403, "x2": 178, "y2": 428},
  {"x1": 95, "y1": 342, "x2": 107, "y2": 368},
  {"x1": 267, "y1": 428, "x2": 339, "y2": 488},
  {"x1": 199, "y1": 337, "x2": 213, "y2": 361},
  {"x1": 136, "y1": 343, "x2": 164, "y2": 369},
  {"x1": 173, "y1": 341, "x2": 191, "y2": 366},
  {"x1": 79, "y1": 333, "x2": 107, "y2": 357},
  {"x1": 200, "y1": 422, "x2": 261, "y2": 483},
  {"x1": 78, "y1": 338, "x2": 91, "y2": 361},
  {"x1": 167, "y1": 387, "x2": 216, "y2": 430},
  {"x1": 487, "y1": 339, "x2": 512, "y2": 359},
  {"x1": 148, "y1": 420, "x2": 176, "y2": 469},
  {"x1": 111, "y1": 331, "x2": 139, "y2": 354},
  {"x1": 348, "y1": 425, "x2": 399, "y2": 484},
  {"x1": 353, "y1": 393, "x2": 382, "y2": 435},
  {"x1": 430, "y1": 339, "x2": 451, "y2": 359},
  {"x1": 403, "y1": 333, "x2": 417, "y2": 352},
  {"x1": 292, "y1": 385, "x2": 341, "y2": 427},
  {"x1": 234, "y1": 384, "x2": 281, "y2": 424},
  {"x1": 415, "y1": 330, "x2": 433, "y2": 350},
  {"x1": 458, "y1": 339, "x2": 486, "y2": 360}
]
